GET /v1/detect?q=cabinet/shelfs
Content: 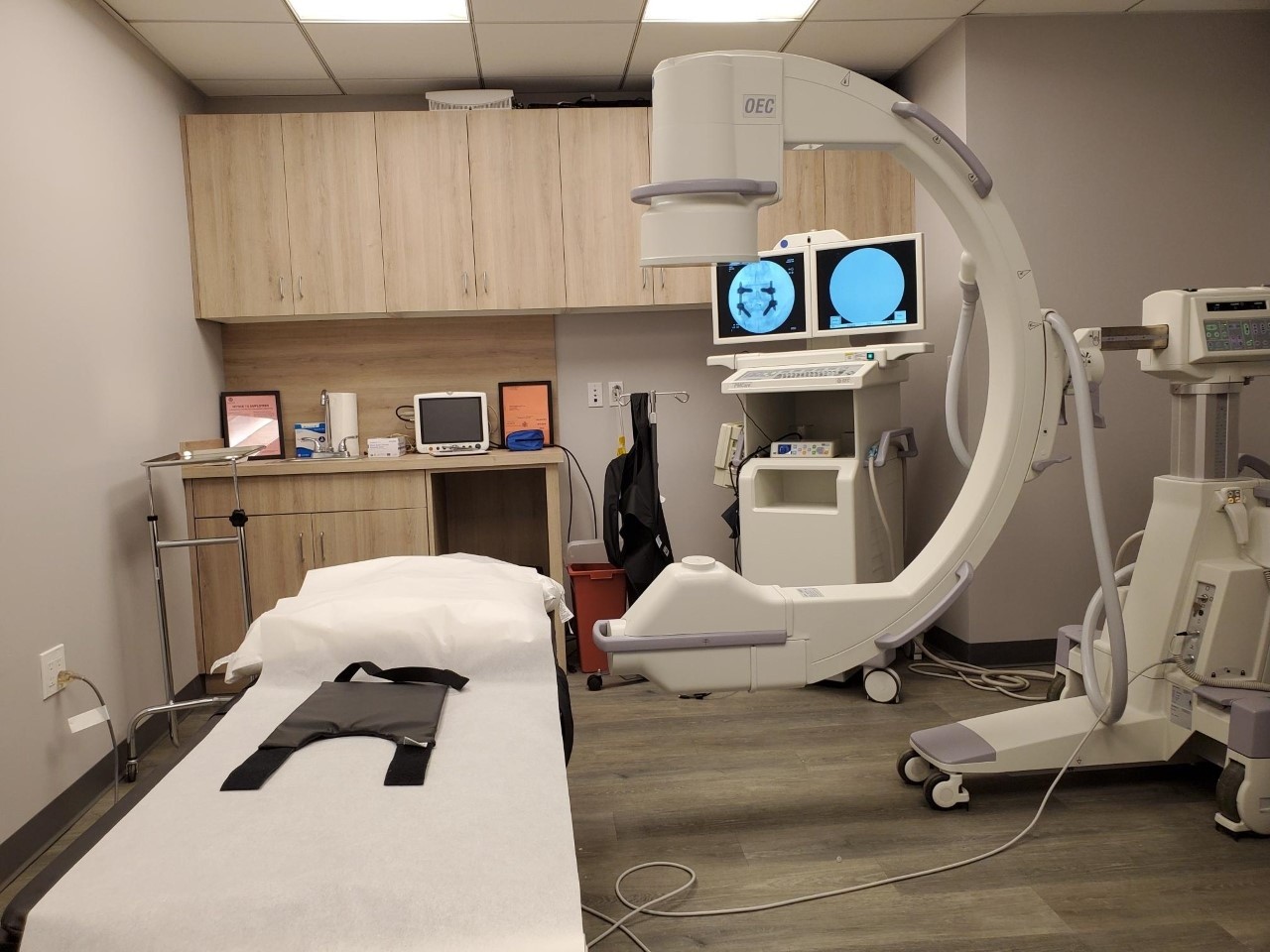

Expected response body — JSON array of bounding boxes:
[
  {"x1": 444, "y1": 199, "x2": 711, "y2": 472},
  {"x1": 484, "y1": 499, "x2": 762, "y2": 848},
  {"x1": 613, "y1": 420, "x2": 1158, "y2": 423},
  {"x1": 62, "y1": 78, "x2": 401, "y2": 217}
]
[
  {"x1": 179, "y1": 105, "x2": 917, "y2": 323},
  {"x1": 195, "y1": 470, "x2": 430, "y2": 674}
]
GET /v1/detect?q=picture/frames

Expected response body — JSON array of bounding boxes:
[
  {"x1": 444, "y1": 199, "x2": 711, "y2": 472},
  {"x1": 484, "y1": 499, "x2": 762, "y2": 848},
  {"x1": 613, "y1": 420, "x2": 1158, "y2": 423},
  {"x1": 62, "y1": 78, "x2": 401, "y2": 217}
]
[
  {"x1": 220, "y1": 390, "x2": 286, "y2": 461},
  {"x1": 497, "y1": 380, "x2": 554, "y2": 449}
]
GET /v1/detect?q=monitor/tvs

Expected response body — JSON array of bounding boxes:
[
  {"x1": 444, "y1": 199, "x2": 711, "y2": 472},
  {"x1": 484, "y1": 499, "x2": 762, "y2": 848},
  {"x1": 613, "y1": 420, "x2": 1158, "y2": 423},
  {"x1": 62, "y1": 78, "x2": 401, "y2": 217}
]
[
  {"x1": 711, "y1": 246, "x2": 813, "y2": 346},
  {"x1": 413, "y1": 392, "x2": 489, "y2": 454},
  {"x1": 809, "y1": 232, "x2": 927, "y2": 337}
]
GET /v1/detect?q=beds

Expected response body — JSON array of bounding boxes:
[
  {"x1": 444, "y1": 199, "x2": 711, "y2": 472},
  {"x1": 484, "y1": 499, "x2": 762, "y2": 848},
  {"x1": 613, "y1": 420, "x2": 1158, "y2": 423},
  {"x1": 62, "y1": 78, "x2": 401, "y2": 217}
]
[{"x1": 19, "y1": 554, "x2": 591, "y2": 952}]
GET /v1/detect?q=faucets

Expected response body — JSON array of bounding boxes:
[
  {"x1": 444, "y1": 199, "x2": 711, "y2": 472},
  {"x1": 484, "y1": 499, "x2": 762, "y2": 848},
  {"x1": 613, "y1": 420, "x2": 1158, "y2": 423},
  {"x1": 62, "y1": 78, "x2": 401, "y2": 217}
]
[{"x1": 319, "y1": 389, "x2": 334, "y2": 457}]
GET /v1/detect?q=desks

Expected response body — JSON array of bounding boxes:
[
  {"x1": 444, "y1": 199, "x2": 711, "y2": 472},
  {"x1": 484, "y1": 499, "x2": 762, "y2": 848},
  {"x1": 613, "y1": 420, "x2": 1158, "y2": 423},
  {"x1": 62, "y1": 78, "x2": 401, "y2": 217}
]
[{"x1": 181, "y1": 437, "x2": 568, "y2": 696}]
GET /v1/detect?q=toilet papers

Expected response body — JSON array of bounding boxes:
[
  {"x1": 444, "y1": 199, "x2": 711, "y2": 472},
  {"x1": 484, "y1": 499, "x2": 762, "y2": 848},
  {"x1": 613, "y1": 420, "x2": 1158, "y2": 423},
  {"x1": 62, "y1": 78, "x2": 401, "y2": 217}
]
[{"x1": 326, "y1": 392, "x2": 360, "y2": 456}]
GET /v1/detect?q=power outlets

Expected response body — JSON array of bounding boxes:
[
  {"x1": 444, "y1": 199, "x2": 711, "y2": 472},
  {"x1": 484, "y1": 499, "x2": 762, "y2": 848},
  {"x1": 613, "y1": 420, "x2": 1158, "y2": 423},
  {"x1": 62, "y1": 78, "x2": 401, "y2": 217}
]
[
  {"x1": 609, "y1": 381, "x2": 625, "y2": 407},
  {"x1": 587, "y1": 382, "x2": 603, "y2": 407},
  {"x1": 40, "y1": 642, "x2": 68, "y2": 701}
]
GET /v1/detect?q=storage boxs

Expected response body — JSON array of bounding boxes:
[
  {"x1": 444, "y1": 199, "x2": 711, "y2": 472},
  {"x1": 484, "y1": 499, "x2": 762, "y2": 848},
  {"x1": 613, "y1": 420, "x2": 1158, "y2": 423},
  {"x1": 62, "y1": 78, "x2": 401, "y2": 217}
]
[{"x1": 425, "y1": 88, "x2": 517, "y2": 111}]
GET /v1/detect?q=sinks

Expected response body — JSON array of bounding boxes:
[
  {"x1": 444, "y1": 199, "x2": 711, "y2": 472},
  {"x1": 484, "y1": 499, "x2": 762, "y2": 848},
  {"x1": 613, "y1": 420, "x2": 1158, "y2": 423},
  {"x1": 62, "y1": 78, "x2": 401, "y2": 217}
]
[{"x1": 282, "y1": 455, "x2": 366, "y2": 461}]
